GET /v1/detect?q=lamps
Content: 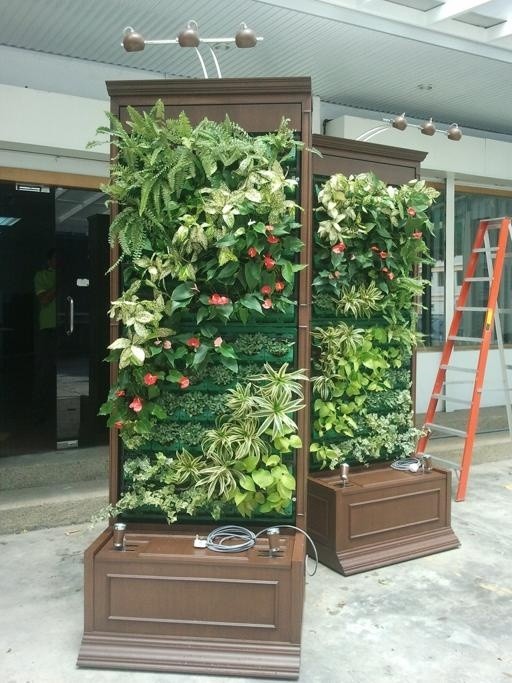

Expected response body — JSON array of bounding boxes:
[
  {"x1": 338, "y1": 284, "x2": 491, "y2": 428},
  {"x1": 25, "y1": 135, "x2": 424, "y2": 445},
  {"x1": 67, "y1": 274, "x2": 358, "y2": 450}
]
[
  {"x1": 120, "y1": 19, "x2": 264, "y2": 80},
  {"x1": 355, "y1": 113, "x2": 464, "y2": 146}
]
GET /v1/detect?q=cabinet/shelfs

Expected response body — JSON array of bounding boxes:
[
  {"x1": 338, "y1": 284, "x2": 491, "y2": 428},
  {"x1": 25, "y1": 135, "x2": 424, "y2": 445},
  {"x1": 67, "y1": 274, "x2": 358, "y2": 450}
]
[
  {"x1": 76, "y1": 78, "x2": 316, "y2": 682},
  {"x1": 309, "y1": 133, "x2": 461, "y2": 578}
]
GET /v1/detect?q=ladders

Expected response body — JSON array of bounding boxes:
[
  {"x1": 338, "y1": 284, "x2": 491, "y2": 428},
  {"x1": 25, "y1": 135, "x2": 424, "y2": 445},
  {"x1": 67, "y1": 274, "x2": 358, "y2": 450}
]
[{"x1": 416, "y1": 216, "x2": 512, "y2": 503}]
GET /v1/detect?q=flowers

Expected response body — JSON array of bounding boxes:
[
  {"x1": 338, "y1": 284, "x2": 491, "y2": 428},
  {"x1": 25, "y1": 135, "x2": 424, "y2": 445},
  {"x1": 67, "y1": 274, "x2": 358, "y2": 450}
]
[
  {"x1": 310, "y1": 195, "x2": 434, "y2": 292},
  {"x1": 94, "y1": 214, "x2": 308, "y2": 447}
]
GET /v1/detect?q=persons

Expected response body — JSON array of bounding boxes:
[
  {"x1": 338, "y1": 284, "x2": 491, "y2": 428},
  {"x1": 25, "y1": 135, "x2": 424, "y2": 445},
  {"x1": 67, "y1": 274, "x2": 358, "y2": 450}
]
[{"x1": 31, "y1": 246, "x2": 66, "y2": 353}]
